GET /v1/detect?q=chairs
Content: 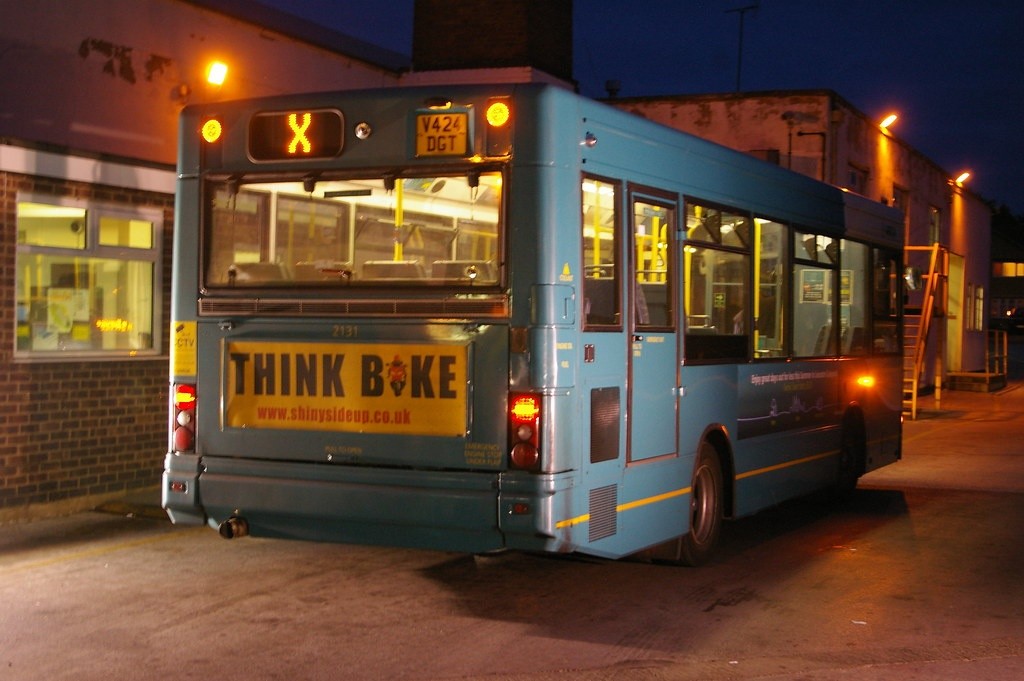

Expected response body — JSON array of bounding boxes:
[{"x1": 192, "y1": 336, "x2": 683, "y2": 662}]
[
  {"x1": 686, "y1": 315, "x2": 717, "y2": 334},
  {"x1": 813, "y1": 325, "x2": 864, "y2": 357},
  {"x1": 585, "y1": 264, "x2": 670, "y2": 326},
  {"x1": 431, "y1": 261, "x2": 496, "y2": 279},
  {"x1": 293, "y1": 261, "x2": 353, "y2": 279},
  {"x1": 234, "y1": 261, "x2": 289, "y2": 280},
  {"x1": 364, "y1": 260, "x2": 426, "y2": 278}
]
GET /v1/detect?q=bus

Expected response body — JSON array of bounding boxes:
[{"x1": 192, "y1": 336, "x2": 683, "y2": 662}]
[{"x1": 161, "y1": 79, "x2": 917, "y2": 567}]
